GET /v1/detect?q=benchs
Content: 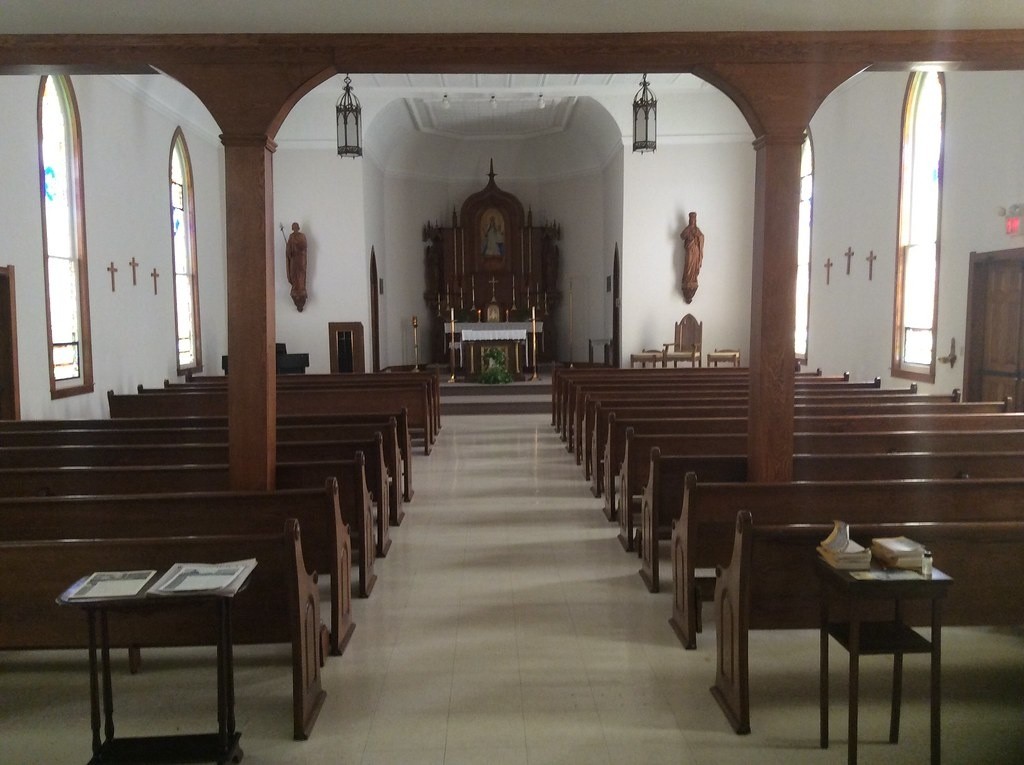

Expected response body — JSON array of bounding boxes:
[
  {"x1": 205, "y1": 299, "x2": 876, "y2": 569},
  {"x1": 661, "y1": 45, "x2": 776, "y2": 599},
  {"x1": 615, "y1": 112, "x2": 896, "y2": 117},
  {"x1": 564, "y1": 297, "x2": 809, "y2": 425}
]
[
  {"x1": 0, "y1": 368, "x2": 440, "y2": 741},
  {"x1": 547, "y1": 360, "x2": 1024, "y2": 737}
]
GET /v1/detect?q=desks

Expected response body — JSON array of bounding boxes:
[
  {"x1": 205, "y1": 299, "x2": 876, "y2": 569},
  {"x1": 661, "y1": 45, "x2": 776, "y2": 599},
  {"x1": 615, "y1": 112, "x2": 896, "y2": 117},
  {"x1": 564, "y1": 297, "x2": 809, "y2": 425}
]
[
  {"x1": 55, "y1": 569, "x2": 256, "y2": 765},
  {"x1": 816, "y1": 558, "x2": 952, "y2": 765},
  {"x1": 461, "y1": 329, "x2": 527, "y2": 383}
]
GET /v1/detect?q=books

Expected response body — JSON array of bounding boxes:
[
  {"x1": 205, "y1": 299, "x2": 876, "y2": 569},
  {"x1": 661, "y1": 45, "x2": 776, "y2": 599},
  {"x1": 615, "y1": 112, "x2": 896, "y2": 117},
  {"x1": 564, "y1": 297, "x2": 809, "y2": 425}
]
[
  {"x1": 816, "y1": 520, "x2": 927, "y2": 569},
  {"x1": 55, "y1": 558, "x2": 258, "y2": 603}
]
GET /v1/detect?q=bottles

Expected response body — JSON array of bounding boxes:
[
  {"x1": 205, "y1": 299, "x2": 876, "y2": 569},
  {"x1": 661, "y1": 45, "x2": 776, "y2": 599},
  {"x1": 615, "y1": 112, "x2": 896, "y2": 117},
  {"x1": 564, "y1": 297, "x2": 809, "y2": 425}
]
[{"x1": 922, "y1": 552, "x2": 933, "y2": 575}]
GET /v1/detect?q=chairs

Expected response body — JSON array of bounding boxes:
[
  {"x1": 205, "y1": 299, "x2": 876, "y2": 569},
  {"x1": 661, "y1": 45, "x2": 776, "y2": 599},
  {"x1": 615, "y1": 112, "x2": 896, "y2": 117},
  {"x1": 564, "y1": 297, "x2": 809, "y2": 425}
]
[{"x1": 662, "y1": 313, "x2": 703, "y2": 367}]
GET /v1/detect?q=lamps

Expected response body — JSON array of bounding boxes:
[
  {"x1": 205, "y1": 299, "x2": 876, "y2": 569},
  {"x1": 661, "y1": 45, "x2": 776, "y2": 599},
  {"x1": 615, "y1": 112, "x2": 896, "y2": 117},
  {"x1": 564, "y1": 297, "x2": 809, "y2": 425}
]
[
  {"x1": 442, "y1": 95, "x2": 450, "y2": 110},
  {"x1": 488, "y1": 96, "x2": 498, "y2": 108},
  {"x1": 335, "y1": 73, "x2": 365, "y2": 161},
  {"x1": 632, "y1": 74, "x2": 658, "y2": 155},
  {"x1": 536, "y1": 95, "x2": 546, "y2": 109}
]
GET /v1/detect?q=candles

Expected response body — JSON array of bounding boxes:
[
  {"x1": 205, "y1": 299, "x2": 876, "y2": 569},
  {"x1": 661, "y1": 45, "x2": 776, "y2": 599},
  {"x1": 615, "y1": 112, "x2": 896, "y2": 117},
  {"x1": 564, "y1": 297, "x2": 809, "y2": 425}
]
[
  {"x1": 506, "y1": 309, "x2": 508, "y2": 320},
  {"x1": 412, "y1": 315, "x2": 416, "y2": 321},
  {"x1": 450, "y1": 308, "x2": 454, "y2": 320},
  {"x1": 478, "y1": 310, "x2": 481, "y2": 319},
  {"x1": 532, "y1": 306, "x2": 535, "y2": 319}
]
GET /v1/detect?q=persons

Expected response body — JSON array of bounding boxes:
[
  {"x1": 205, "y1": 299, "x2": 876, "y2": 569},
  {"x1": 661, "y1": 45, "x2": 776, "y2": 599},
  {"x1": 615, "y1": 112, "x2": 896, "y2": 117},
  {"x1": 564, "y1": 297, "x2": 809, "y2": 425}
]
[
  {"x1": 682, "y1": 212, "x2": 704, "y2": 304},
  {"x1": 285, "y1": 222, "x2": 308, "y2": 313}
]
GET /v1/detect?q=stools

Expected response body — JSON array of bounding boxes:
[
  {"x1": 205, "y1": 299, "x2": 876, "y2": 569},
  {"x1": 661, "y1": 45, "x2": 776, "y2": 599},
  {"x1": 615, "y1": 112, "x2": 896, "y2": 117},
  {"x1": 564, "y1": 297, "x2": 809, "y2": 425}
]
[
  {"x1": 629, "y1": 348, "x2": 666, "y2": 368},
  {"x1": 707, "y1": 348, "x2": 740, "y2": 368}
]
